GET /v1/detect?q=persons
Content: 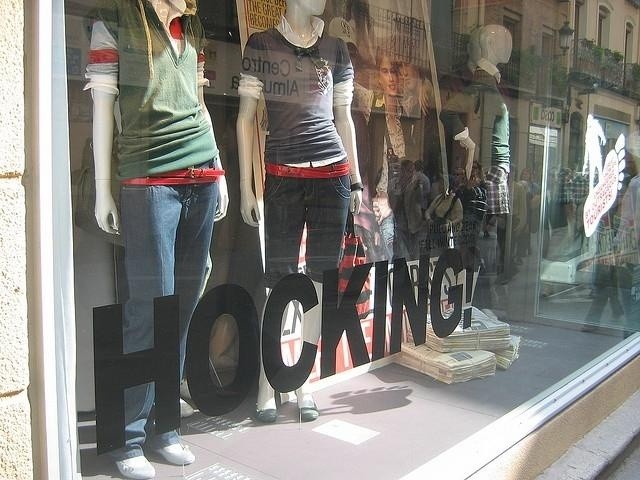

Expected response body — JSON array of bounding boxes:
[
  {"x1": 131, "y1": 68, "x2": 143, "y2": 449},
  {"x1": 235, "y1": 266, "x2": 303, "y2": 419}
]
[
  {"x1": 375, "y1": 147, "x2": 640, "y2": 341},
  {"x1": 329, "y1": 15, "x2": 444, "y2": 263},
  {"x1": 237, "y1": 0, "x2": 363, "y2": 425},
  {"x1": 429, "y1": 25, "x2": 512, "y2": 311},
  {"x1": 80, "y1": 0, "x2": 229, "y2": 479}
]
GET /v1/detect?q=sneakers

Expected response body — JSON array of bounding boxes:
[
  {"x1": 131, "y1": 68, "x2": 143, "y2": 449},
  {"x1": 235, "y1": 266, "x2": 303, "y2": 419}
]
[
  {"x1": 116, "y1": 457, "x2": 155, "y2": 479},
  {"x1": 256, "y1": 404, "x2": 278, "y2": 422},
  {"x1": 298, "y1": 402, "x2": 319, "y2": 421},
  {"x1": 155, "y1": 446, "x2": 194, "y2": 465}
]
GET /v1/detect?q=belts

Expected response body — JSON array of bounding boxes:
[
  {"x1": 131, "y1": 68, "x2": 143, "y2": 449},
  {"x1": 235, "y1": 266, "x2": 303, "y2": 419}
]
[
  {"x1": 265, "y1": 163, "x2": 349, "y2": 179},
  {"x1": 122, "y1": 169, "x2": 225, "y2": 185}
]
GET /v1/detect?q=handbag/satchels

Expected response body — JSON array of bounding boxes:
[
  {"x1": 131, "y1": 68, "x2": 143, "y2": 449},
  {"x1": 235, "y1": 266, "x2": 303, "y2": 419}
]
[{"x1": 429, "y1": 216, "x2": 448, "y2": 241}]
